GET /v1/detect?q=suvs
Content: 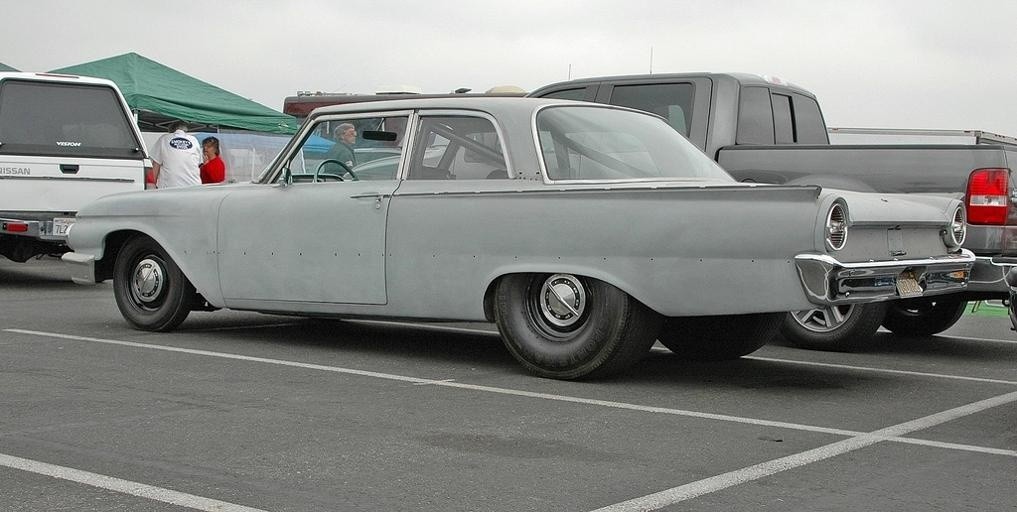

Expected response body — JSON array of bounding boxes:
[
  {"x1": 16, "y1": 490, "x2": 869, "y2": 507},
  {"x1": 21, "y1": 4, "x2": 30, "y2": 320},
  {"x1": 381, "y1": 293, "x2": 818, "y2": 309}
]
[{"x1": 0, "y1": 72, "x2": 153, "y2": 273}]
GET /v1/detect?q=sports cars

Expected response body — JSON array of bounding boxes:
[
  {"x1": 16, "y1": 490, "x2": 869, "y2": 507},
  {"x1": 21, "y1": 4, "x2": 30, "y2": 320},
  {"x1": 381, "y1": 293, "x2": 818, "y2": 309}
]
[{"x1": 59, "y1": 93, "x2": 974, "y2": 384}]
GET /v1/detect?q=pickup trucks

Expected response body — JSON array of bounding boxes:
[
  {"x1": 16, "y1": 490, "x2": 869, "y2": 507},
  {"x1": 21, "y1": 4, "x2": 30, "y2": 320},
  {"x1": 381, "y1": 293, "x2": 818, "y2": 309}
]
[{"x1": 342, "y1": 72, "x2": 1016, "y2": 344}]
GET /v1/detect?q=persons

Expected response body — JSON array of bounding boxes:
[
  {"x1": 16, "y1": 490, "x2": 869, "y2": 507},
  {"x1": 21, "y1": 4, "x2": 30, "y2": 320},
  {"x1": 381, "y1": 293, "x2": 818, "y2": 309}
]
[
  {"x1": 148, "y1": 121, "x2": 202, "y2": 191},
  {"x1": 196, "y1": 134, "x2": 225, "y2": 183},
  {"x1": 322, "y1": 122, "x2": 357, "y2": 175}
]
